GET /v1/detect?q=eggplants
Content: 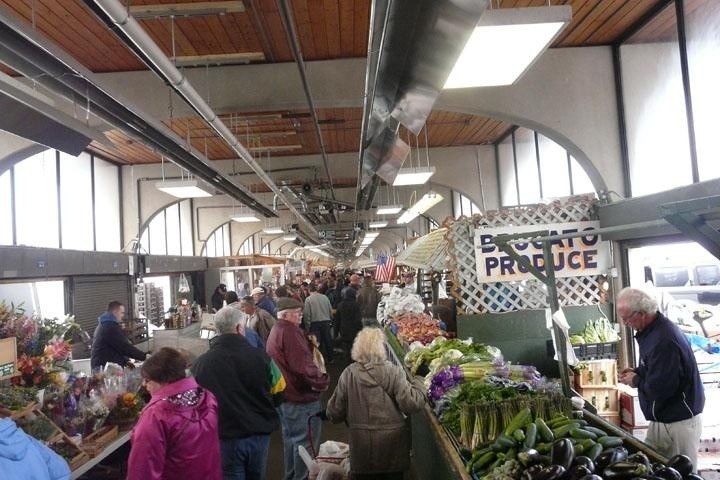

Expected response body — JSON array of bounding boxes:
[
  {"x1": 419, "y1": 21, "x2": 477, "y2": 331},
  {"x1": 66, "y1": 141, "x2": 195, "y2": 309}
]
[{"x1": 485, "y1": 438, "x2": 703, "y2": 480}]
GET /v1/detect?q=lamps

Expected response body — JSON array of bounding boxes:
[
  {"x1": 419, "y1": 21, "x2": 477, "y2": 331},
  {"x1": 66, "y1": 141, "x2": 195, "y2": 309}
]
[
  {"x1": 218, "y1": 112, "x2": 282, "y2": 223},
  {"x1": 370, "y1": 206, "x2": 387, "y2": 230},
  {"x1": 249, "y1": 144, "x2": 301, "y2": 233},
  {"x1": 397, "y1": 192, "x2": 443, "y2": 227},
  {"x1": 444, "y1": 3, "x2": 571, "y2": 89},
  {"x1": 353, "y1": 229, "x2": 379, "y2": 257},
  {"x1": 391, "y1": 116, "x2": 433, "y2": 190},
  {"x1": 134, "y1": 2, "x2": 247, "y2": 197},
  {"x1": 376, "y1": 179, "x2": 401, "y2": 217},
  {"x1": 274, "y1": 183, "x2": 301, "y2": 241}
]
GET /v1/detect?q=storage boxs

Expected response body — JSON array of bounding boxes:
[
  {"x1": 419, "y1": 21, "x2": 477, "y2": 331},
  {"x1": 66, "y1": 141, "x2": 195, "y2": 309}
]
[
  {"x1": 621, "y1": 423, "x2": 649, "y2": 442},
  {"x1": 616, "y1": 383, "x2": 656, "y2": 426}
]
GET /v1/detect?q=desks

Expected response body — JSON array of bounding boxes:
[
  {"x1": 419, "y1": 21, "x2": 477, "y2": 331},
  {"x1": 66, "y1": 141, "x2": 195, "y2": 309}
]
[{"x1": 70, "y1": 427, "x2": 132, "y2": 479}]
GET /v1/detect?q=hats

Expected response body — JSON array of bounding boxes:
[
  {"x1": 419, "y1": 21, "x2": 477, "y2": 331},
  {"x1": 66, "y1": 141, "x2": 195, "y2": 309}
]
[
  {"x1": 276, "y1": 296, "x2": 304, "y2": 310},
  {"x1": 251, "y1": 287, "x2": 265, "y2": 296}
]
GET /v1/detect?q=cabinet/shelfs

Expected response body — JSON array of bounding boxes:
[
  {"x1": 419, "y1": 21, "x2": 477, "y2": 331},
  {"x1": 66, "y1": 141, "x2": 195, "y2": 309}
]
[
  {"x1": 417, "y1": 267, "x2": 432, "y2": 316},
  {"x1": 137, "y1": 282, "x2": 147, "y2": 318},
  {"x1": 146, "y1": 283, "x2": 165, "y2": 326}
]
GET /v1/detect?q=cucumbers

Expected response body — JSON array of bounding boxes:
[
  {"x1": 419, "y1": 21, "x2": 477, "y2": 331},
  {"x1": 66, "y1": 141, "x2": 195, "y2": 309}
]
[{"x1": 466, "y1": 408, "x2": 624, "y2": 474}]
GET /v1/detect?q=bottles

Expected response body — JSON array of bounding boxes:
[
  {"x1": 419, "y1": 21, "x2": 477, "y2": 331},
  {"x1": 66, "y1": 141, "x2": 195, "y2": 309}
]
[{"x1": 165, "y1": 299, "x2": 203, "y2": 330}]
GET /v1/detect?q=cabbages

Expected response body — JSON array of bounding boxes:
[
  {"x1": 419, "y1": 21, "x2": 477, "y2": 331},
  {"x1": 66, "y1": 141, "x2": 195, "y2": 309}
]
[{"x1": 568, "y1": 315, "x2": 620, "y2": 345}]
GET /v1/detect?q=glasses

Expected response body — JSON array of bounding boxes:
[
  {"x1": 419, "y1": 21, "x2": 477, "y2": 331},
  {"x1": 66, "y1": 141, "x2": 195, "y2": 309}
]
[
  {"x1": 285, "y1": 309, "x2": 303, "y2": 315},
  {"x1": 144, "y1": 377, "x2": 154, "y2": 384},
  {"x1": 620, "y1": 310, "x2": 638, "y2": 325}
]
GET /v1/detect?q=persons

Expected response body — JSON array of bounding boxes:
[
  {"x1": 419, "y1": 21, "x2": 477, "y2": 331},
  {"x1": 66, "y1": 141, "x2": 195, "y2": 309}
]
[
  {"x1": 211, "y1": 268, "x2": 378, "y2": 362},
  {"x1": 195, "y1": 307, "x2": 287, "y2": 480},
  {"x1": 0, "y1": 417, "x2": 71, "y2": 479},
  {"x1": 91, "y1": 302, "x2": 146, "y2": 373},
  {"x1": 616, "y1": 288, "x2": 705, "y2": 472},
  {"x1": 324, "y1": 327, "x2": 429, "y2": 479},
  {"x1": 128, "y1": 348, "x2": 221, "y2": 480},
  {"x1": 267, "y1": 298, "x2": 330, "y2": 480}
]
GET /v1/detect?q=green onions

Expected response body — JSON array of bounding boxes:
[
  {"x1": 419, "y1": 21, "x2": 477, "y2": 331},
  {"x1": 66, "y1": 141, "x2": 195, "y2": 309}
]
[{"x1": 458, "y1": 395, "x2": 569, "y2": 450}]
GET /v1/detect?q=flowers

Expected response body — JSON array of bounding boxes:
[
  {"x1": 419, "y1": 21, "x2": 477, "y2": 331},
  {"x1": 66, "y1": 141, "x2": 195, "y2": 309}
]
[{"x1": 0, "y1": 297, "x2": 143, "y2": 433}]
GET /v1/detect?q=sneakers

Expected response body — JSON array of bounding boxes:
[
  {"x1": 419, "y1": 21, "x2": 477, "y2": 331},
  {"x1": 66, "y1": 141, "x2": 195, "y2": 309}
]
[{"x1": 326, "y1": 358, "x2": 336, "y2": 364}]
